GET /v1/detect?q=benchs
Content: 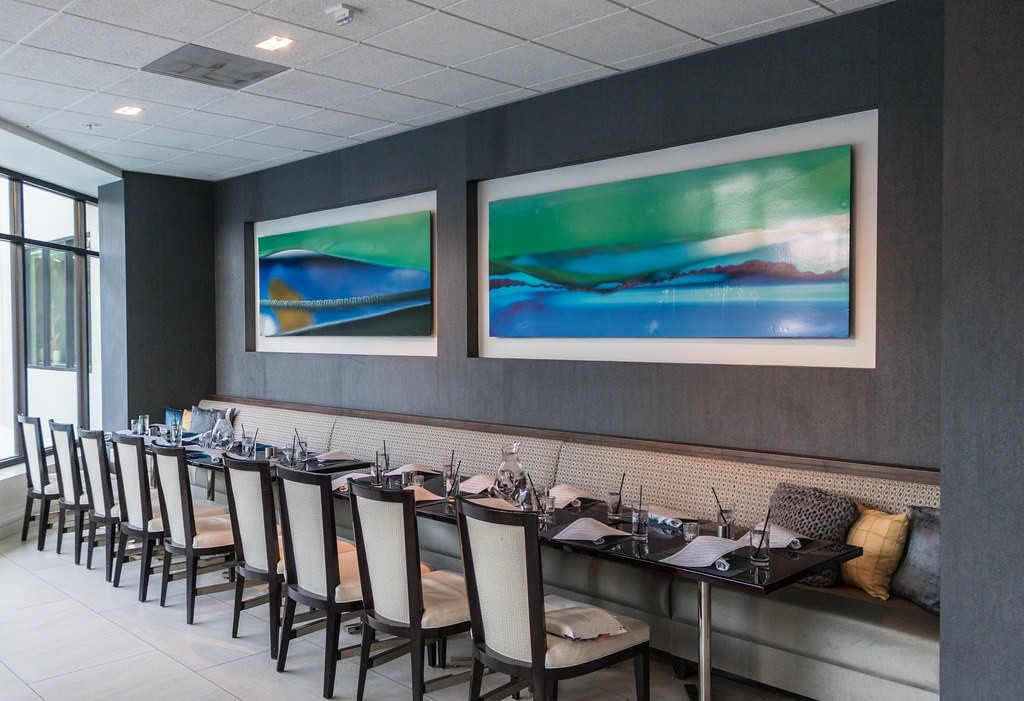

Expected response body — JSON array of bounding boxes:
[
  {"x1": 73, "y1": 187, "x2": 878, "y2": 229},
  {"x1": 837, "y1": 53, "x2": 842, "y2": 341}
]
[{"x1": 198, "y1": 395, "x2": 941, "y2": 701}]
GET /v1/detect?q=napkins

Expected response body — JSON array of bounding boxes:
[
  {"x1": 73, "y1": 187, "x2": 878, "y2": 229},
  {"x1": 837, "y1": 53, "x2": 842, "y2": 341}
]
[
  {"x1": 715, "y1": 556, "x2": 730, "y2": 571},
  {"x1": 211, "y1": 458, "x2": 221, "y2": 463},
  {"x1": 317, "y1": 458, "x2": 324, "y2": 462},
  {"x1": 105, "y1": 436, "x2": 112, "y2": 441},
  {"x1": 791, "y1": 539, "x2": 802, "y2": 549},
  {"x1": 159, "y1": 428, "x2": 169, "y2": 435},
  {"x1": 341, "y1": 484, "x2": 348, "y2": 492},
  {"x1": 594, "y1": 538, "x2": 605, "y2": 544},
  {"x1": 488, "y1": 485, "x2": 493, "y2": 492},
  {"x1": 571, "y1": 498, "x2": 580, "y2": 507}
]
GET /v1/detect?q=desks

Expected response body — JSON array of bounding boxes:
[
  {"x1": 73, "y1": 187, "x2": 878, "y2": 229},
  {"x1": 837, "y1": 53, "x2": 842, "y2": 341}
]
[{"x1": 104, "y1": 425, "x2": 864, "y2": 701}]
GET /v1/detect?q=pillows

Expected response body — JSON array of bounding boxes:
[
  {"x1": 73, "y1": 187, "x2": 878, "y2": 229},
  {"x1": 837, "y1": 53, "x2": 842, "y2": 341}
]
[
  {"x1": 767, "y1": 482, "x2": 941, "y2": 615},
  {"x1": 164, "y1": 406, "x2": 236, "y2": 433}
]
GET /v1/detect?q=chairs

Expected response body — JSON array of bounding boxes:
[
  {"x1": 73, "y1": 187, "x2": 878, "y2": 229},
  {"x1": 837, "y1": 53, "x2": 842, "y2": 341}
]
[{"x1": 17, "y1": 413, "x2": 650, "y2": 701}]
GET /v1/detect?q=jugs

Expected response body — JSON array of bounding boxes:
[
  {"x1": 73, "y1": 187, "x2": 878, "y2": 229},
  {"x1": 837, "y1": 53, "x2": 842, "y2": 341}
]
[
  {"x1": 212, "y1": 407, "x2": 234, "y2": 453},
  {"x1": 493, "y1": 442, "x2": 528, "y2": 508}
]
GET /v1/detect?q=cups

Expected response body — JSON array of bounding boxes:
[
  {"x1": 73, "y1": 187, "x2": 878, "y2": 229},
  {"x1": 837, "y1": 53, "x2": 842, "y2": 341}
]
[
  {"x1": 631, "y1": 500, "x2": 651, "y2": 541},
  {"x1": 286, "y1": 436, "x2": 308, "y2": 470},
  {"x1": 131, "y1": 414, "x2": 157, "y2": 437},
  {"x1": 750, "y1": 522, "x2": 772, "y2": 566},
  {"x1": 607, "y1": 483, "x2": 623, "y2": 520},
  {"x1": 683, "y1": 522, "x2": 700, "y2": 544},
  {"x1": 169, "y1": 420, "x2": 182, "y2": 446},
  {"x1": 402, "y1": 470, "x2": 425, "y2": 487},
  {"x1": 265, "y1": 447, "x2": 277, "y2": 460},
  {"x1": 717, "y1": 501, "x2": 736, "y2": 540},
  {"x1": 242, "y1": 432, "x2": 256, "y2": 461},
  {"x1": 370, "y1": 447, "x2": 389, "y2": 486},
  {"x1": 442, "y1": 458, "x2": 460, "y2": 503},
  {"x1": 531, "y1": 483, "x2": 556, "y2": 513}
]
